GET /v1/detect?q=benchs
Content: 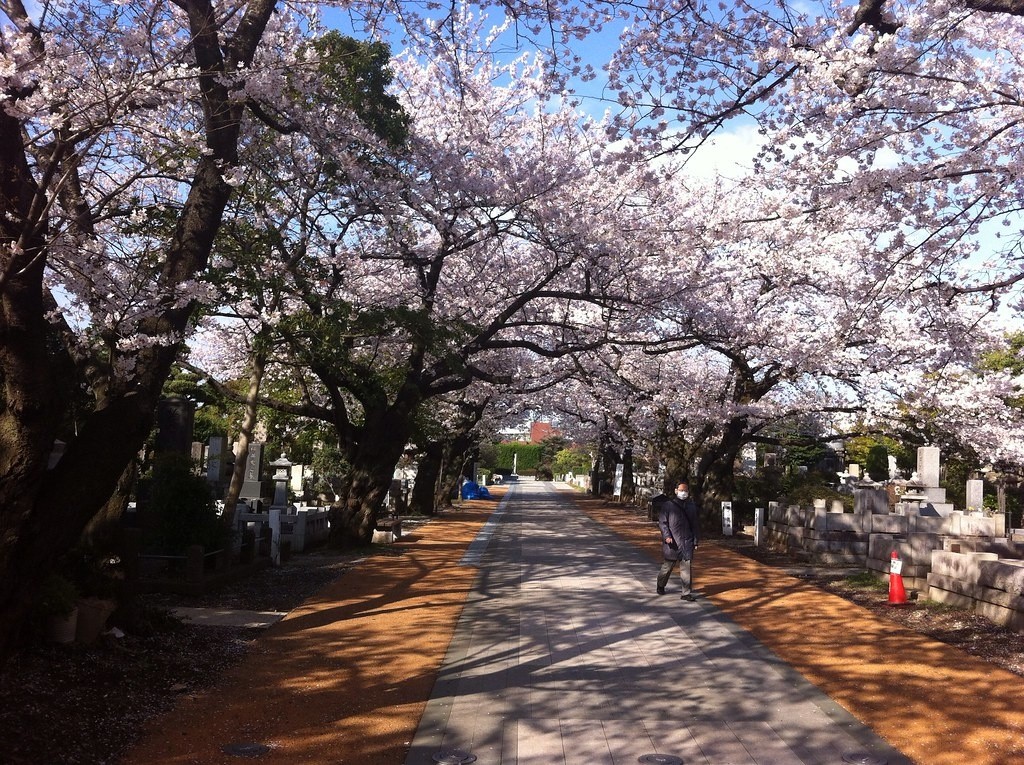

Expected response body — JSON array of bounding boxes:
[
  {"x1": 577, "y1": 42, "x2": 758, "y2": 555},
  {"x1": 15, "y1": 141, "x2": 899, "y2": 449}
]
[{"x1": 371, "y1": 518, "x2": 404, "y2": 543}]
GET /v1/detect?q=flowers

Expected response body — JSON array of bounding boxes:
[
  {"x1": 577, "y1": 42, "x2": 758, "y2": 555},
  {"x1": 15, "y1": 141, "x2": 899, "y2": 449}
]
[
  {"x1": 967, "y1": 506, "x2": 975, "y2": 511},
  {"x1": 978, "y1": 506, "x2": 993, "y2": 517}
]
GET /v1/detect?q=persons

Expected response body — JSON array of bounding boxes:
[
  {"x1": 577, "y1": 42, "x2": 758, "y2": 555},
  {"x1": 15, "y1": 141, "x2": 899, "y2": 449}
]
[{"x1": 656, "y1": 482, "x2": 700, "y2": 601}]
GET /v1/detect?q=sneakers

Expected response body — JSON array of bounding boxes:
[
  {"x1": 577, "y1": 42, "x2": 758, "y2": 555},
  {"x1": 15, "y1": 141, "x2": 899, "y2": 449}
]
[
  {"x1": 657, "y1": 582, "x2": 664, "y2": 595},
  {"x1": 681, "y1": 594, "x2": 695, "y2": 601}
]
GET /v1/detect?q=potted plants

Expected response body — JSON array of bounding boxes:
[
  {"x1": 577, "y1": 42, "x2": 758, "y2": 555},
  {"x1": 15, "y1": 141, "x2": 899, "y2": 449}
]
[
  {"x1": 33, "y1": 574, "x2": 82, "y2": 647},
  {"x1": 72, "y1": 567, "x2": 121, "y2": 645}
]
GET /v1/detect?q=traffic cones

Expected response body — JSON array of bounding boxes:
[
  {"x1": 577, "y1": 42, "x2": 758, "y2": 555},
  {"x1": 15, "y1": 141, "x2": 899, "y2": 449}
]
[{"x1": 881, "y1": 550, "x2": 916, "y2": 607}]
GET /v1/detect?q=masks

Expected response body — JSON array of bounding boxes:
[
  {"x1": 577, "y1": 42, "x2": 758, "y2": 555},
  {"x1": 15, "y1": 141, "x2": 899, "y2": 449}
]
[{"x1": 677, "y1": 491, "x2": 688, "y2": 500}]
